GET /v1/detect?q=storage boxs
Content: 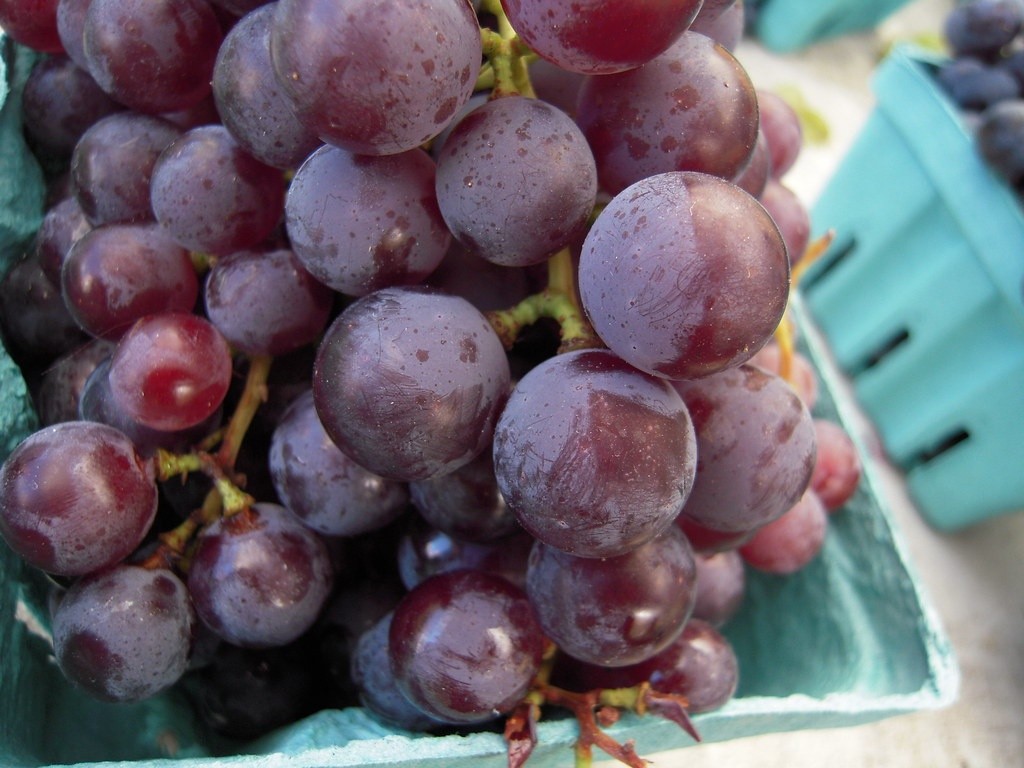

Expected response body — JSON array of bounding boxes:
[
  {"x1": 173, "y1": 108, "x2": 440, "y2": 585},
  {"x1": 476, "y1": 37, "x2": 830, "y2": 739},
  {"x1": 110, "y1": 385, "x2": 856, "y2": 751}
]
[
  {"x1": 793, "y1": 41, "x2": 1024, "y2": 533},
  {"x1": 0, "y1": 0, "x2": 963, "y2": 767}
]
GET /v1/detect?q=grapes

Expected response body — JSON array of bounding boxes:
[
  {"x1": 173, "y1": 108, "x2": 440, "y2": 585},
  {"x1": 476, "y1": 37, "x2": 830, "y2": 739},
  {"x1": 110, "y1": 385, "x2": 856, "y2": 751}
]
[{"x1": 0, "y1": 0, "x2": 1024, "y2": 733}]
